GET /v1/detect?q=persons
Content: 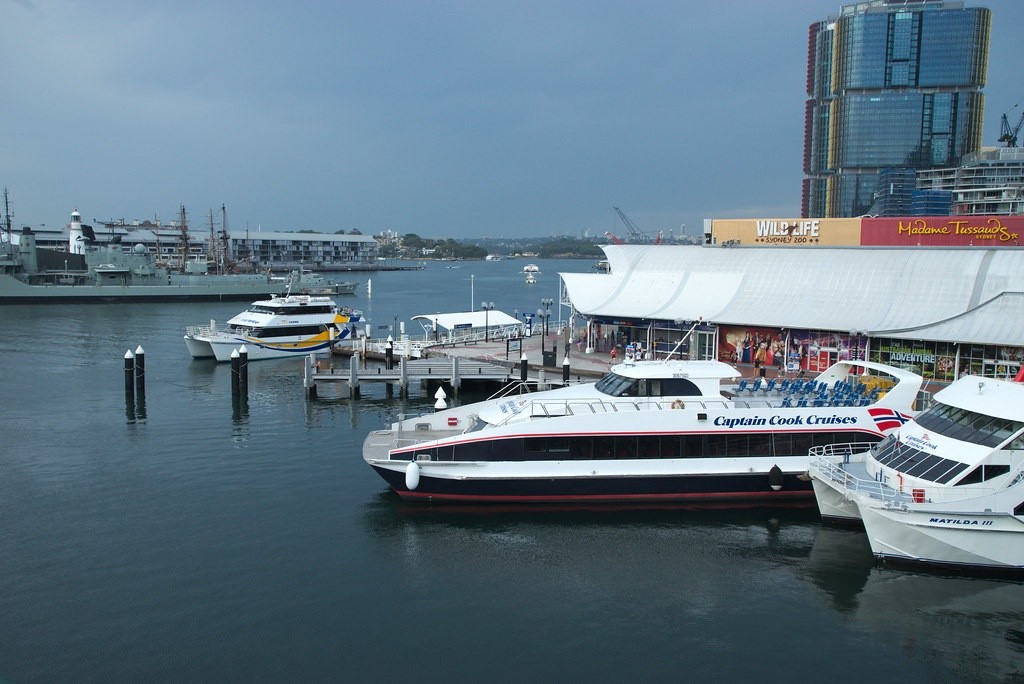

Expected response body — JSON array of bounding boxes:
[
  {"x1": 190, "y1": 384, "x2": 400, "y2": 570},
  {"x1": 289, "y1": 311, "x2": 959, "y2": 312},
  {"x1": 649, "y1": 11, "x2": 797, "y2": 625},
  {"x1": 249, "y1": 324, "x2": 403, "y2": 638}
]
[
  {"x1": 729, "y1": 351, "x2": 738, "y2": 366},
  {"x1": 660, "y1": 337, "x2": 663, "y2": 342},
  {"x1": 938, "y1": 357, "x2": 953, "y2": 371},
  {"x1": 778, "y1": 366, "x2": 785, "y2": 384},
  {"x1": 576, "y1": 338, "x2": 582, "y2": 351},
  {"x1": 352, "y1": 324, "x2": 357, "y2": 338},
  {"x1": 754, "y1": 358, "x2": 760, "y2": 377},
  {"x1": 830, "y1": 338, "x2": 838, "y2": 358},
  {"x1": 582, "y1": 329, "x2": 587, "y2": 344},
  {"x1": 340, "y1": 306, "x2": 343, "y2": 312},
  {"x1": 610, "y1": 330, "x2": 615, "y2": 346},
  {"x1": 796, "y1": 365, "x2": 803, "y2": 378},
  {"x1": 959, "y1": 366, "x2": 968, "y2": 379},
  {"x1": 609, "y1": 347, "x2": 617, "y2": 364},
  {"x1": 851, "y1": 340, "x2": 864, "y2": 360},
  {"x1": 742, "y1": 331, "x2": 785, "y2": 366},
  {"x1": 564, "y1": 341, "x2": 570, "y2": 358},
  {"x1": 790, "y1": 336, "x2": 805, "y2": 357},
  {"x1": 629, "y1": 341, "x2": 642, "y2": 361}
]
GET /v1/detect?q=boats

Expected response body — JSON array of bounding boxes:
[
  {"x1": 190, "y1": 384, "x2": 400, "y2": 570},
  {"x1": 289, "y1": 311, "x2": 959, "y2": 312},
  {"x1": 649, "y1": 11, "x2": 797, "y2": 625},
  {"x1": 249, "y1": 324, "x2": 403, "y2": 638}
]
[
  {"x1": 0, "y1": 186, "x2": 360, "y2": 297},
  {"x1": 523, "y1": 263, "x2": 540, "y2": 272},
  {"x1": 526, "y1": 271, "x2": 536, "y2": 284},
  {"x1": 184, "y1": 324, "x2": 227, "y2": 359},
  {"x1": 796, "y1": 361, "x2": 1024, "y2": 571},
  {"x1": 193, "y1": 270, "x2": 366, "y2": 362},
  {"x1": 362, "y1": 356, "x2": 931, "y2": 514}
]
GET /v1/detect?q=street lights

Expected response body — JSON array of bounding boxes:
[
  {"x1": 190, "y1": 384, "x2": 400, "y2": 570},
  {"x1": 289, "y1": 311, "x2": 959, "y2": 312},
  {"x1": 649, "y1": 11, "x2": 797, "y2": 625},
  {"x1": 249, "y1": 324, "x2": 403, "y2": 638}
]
[
  {"x1": 538, "y1": 309, "x2": 552, "y2": 354},
  {"x1": 481, "y1": 302, "x2": 495, "y2": 343},
  {"x1": 514, "y1": 309, "x2": 519, "y2": 321},
  {"x1": 470, "y1": 274, "x2": 475, "y2": 312},
  {"x1": 541, "y1": 298, "x2": 554, "y2": 336}
]
